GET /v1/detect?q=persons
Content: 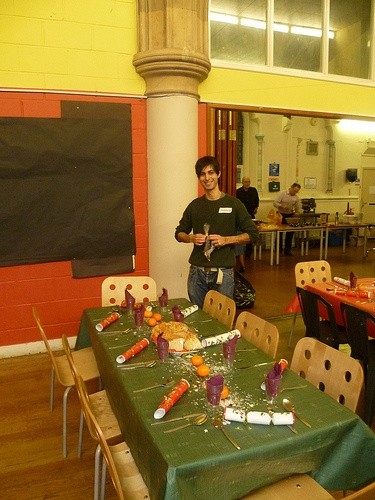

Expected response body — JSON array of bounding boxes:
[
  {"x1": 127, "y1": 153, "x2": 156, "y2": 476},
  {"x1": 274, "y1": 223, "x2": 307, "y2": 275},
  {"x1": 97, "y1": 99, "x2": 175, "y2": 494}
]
[
  {"x1": 274, "y1": 182, "x2": 301, "y2": 256},
  {"x1": 236, "y1": 177, "x2": 259, "y2": 260},
  {"x1": 175, "y1": 156, "x2": 260, "y2": 309}
]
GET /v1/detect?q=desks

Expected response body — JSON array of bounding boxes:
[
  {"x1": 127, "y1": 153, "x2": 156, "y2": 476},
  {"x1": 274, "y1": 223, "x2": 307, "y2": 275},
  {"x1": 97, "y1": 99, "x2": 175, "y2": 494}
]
[{"x1": 242, "y1": 216, "x2": 375, "y2": 266}]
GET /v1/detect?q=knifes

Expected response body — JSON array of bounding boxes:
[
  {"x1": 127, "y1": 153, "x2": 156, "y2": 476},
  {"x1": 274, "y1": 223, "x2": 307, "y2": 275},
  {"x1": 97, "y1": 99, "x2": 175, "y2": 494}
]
[
  {"x1": 238, "y1": 361, "x2": 277, "y2": 370},
  {"x1": 150, "y1": 414, "x2": 202, "y2": 426}
]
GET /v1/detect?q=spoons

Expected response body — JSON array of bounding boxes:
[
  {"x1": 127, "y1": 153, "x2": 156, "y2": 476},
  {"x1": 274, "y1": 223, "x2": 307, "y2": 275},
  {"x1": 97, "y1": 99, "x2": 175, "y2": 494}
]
[
  {"x1": 282, "y1": 399, "x2": 311, "y2": 428},
  {"x1": 163, "y1": 414, "x2": 208, "y2": 433}
]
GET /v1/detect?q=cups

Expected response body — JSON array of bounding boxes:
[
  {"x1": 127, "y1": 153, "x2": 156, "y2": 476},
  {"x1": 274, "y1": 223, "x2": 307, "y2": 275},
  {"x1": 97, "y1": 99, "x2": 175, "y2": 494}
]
[
  {"x1": 357, "y1": 283, "x2": 365, "y2": 293},
  {"x1": 157, "y1": 341, "x2": 168, "y2": 360},
  {"x1": 125, "y1": 299, "x2": 184, "y2": 327},
  {"x1": 205, "y1": 379, "x2": 224, "y2": 404},
  {"x1": 222, "y1": 344, "x2": 236, "y2": 367},
  {"x1": 349, "y1": 277, "x2": 358, "y2": 291},
  {"x1": 264, "y1": 371, "x2": 283, "y2": 400}
]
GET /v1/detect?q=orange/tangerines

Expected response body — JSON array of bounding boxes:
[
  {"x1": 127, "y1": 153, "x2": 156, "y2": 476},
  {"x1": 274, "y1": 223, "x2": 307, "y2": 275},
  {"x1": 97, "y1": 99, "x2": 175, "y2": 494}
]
[
  {"x1": 152, "y1": 314, "x2": 161, "y2": 321},
  {"x1": 221, "y1": 386, "x2": 228, "y2": 399},
  {"x1": 148, "y1": 318, "x2": 156, "y2": 326},
  {"x1": 192, "y1": 355, "x2": 203, "y2": 366},
  {"x1": 144, "y1": 311, "x2": 153, "y2": 318},
  {"x1": 196, "y1": 365, "x2": 211, "y2": 376}
]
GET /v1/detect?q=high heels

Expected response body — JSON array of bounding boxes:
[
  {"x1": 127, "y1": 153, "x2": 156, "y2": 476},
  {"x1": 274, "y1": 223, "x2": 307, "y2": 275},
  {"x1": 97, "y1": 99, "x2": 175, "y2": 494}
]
[{"x1": 240, "y1": 267, "x2": 247, "y2": 272}]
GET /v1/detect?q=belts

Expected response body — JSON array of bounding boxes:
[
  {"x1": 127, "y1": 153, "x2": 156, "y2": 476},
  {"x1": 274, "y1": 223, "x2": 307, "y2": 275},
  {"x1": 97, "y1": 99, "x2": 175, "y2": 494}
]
[{"x1": 199, "y1": 266, "x2": 227, "y2": 284}]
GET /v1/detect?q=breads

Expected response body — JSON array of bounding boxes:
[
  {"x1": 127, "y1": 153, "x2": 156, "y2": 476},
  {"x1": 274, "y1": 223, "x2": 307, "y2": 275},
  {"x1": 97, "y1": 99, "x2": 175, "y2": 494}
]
[{"x1": 153, "y1": 321, "x2": 201, "y2": 351}]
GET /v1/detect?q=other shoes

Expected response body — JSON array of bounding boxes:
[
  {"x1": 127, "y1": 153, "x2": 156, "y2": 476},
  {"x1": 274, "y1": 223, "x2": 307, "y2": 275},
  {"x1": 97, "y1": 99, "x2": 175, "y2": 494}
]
[{"x1": 285, "y1": 252, "x2": 293, "y2": 255}]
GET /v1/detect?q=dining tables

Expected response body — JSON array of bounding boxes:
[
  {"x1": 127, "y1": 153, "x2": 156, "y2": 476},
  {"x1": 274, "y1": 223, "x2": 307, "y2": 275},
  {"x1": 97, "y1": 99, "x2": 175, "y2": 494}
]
[
  {"x1": 75, "y1": 298, "x2": 375, "y2": 500},
  {"x1": 284, "y1": 277, "x2": 375, "y2": 342}
]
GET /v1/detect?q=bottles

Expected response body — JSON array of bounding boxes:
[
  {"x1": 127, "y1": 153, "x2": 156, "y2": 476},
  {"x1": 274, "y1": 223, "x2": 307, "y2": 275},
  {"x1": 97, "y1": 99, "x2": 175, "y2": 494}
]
[{"x1": 335, "y1": 212, "x2": 339, "y2": 225}]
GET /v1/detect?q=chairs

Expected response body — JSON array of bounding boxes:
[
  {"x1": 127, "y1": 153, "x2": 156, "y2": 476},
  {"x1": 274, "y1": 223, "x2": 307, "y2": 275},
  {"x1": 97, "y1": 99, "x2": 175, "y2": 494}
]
[
  {"x1": 100, "y1": 276, "x2": 159, "y2": 310},
  {"x1": 200, "y1": 258, "x2": 364, "y2": 412},
  {"x1": 30, "y1": 306, "x2": 152, "y2": 499},
  {"x1": 238, "y1": 472, "x2": 375, "y2": 500}
]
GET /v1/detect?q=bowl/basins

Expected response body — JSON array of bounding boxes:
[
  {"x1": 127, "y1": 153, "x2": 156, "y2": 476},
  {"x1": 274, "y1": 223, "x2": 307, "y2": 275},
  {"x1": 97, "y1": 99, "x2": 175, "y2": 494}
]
[
  {"x1": 343, "y1": 215, "x2": 358, "y2": 225},
  {"x1": 285, "y1": 218, "x2": 300, "y2": 224}
]
